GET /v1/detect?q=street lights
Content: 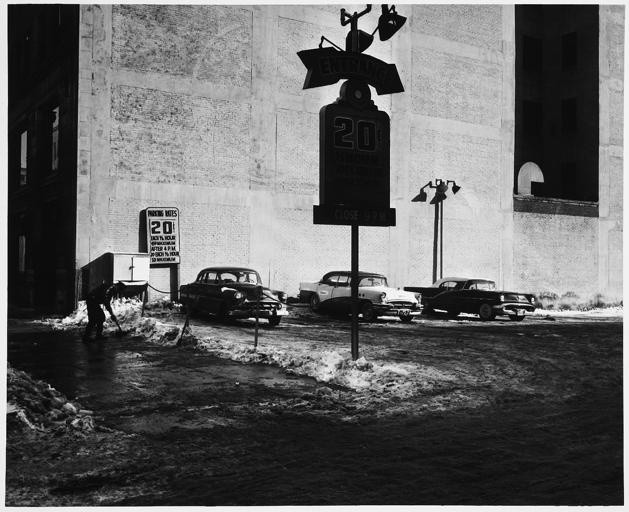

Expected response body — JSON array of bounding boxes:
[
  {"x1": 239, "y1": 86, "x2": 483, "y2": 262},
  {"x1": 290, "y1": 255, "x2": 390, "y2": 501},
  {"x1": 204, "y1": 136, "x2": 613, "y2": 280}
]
[
  {"x1": 339, "y1": 6, "x2": 407, "y2": 358},
  {"x1": 412, "y1": 179, "x2": 460, "y2": 282}
]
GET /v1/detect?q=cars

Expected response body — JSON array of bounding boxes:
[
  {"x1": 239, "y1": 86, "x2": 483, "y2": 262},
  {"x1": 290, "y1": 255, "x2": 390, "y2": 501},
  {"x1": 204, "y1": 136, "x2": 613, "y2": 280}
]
[
  {"x1": 298, "y1": 272, "x2": 424, "y2": 323},
  {"x1": 180, "y1": 268, "x2": 287, "y2": 324}
]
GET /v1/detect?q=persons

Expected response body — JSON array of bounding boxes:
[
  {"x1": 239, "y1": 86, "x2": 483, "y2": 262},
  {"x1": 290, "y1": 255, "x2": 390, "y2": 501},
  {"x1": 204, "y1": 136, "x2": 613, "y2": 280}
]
[{"x1": 82, "y1": 281, "x2": 126, "y2": 340}]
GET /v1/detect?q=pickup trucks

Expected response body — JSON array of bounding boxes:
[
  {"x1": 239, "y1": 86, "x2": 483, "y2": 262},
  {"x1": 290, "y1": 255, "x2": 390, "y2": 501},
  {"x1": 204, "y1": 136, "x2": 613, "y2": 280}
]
[{"x1": 404, "y1": 276, "x2": 536, "y2": 322}]
